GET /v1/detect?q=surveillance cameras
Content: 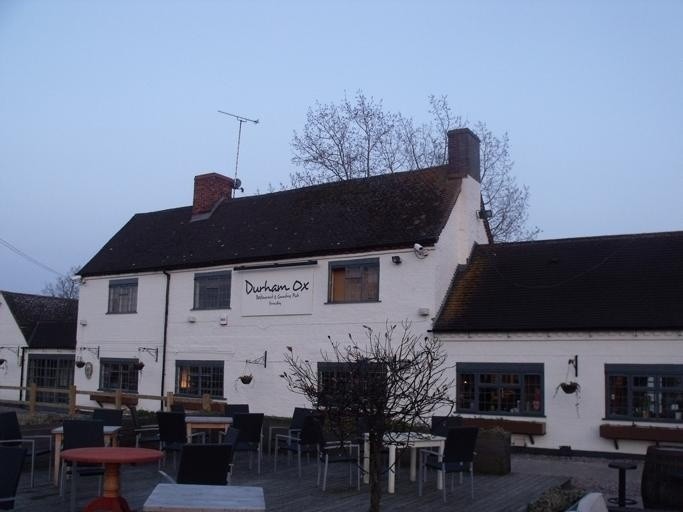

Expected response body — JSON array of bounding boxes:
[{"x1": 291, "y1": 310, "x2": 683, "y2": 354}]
[
  {"x1": 71, "y1": 275, "x2": 82, "y2": 283},
  {"x1": 414, "y1": 243, "x2": 423, "y2": 253}
]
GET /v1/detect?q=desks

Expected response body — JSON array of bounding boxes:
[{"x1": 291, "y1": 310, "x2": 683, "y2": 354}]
[
  {"x1": 51, "y1": 425, "x2": 123, "y2": 488},
  {"x1": 60, "y1": 446, "x2": 165, "y2": 512},
  {"x1": 362, "y1": 431, "x2": 447, "y2": 494},
  {"x1": 143, "y1": 483, "x2": 266, "y2": 512}
]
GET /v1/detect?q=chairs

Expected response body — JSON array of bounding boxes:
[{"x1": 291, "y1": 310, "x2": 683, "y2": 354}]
[
  {"x1": 353, "y1": 414, "x2": 401, "y2": 480},
  {"x1": 417, "y1": 426, "x2": 479, "y2": 505},
  {"x1": 422, "y1": 416, "x2": 464, "y2": 483},
  {"x1": 0, "y1": 411, "x2": 108, "y2": 512},
  {"x1": 156, "y1": 402, "x2": 327, "y2": 479},
  {"x1": 158, "y1": 443, "x2": 234, "y2": 485},
  {"x1": 93, "y1": 409, "x2": 123, "y2": 426},
  {"x1": 306, "y1": 415, "x2": 361, "y2": 492}
]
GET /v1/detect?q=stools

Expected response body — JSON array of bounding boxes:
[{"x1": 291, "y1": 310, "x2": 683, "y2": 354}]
[{"x1": 605, "y1": 458, "x2": 644, "y2": 512}]
